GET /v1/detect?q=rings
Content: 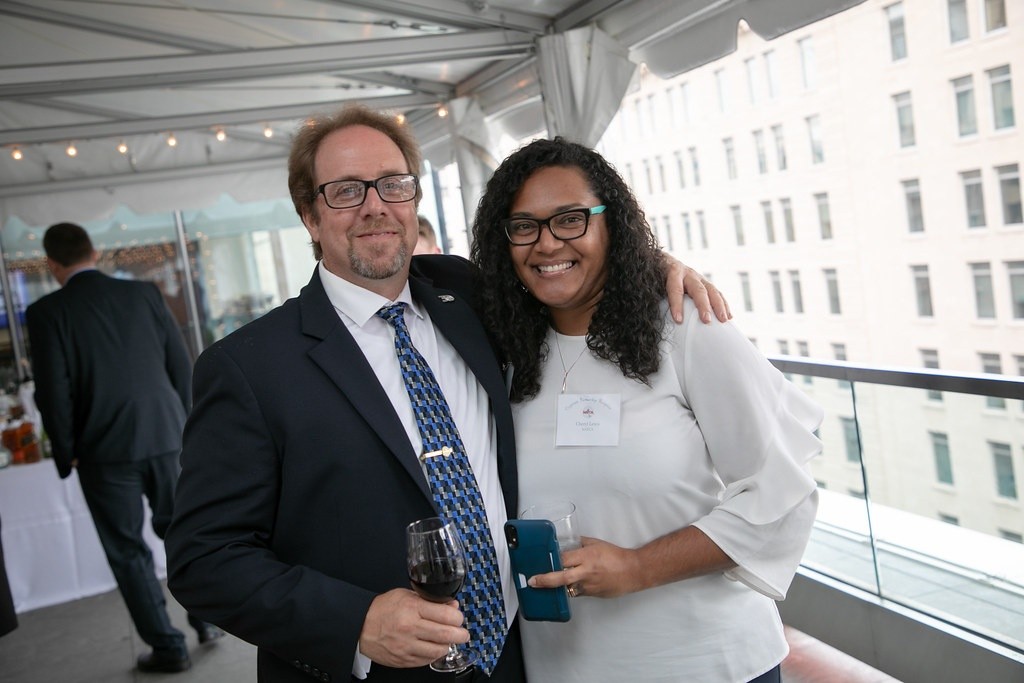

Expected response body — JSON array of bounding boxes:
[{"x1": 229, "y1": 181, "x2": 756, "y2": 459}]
[{"x1": 567, "y1": 584, "x2": 576, "y2": 597}]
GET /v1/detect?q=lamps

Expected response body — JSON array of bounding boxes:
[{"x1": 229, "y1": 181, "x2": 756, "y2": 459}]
[{"x1": 11, "y1": 105, "x2": 448, "y2": 160}]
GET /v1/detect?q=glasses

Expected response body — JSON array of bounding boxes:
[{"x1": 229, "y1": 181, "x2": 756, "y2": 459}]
[
  {"x1": 503, "y1": 205, "x2": 608, "y2": 246},
  {"x1": 313, "y1": 174, "x2": 419, "y2": 209}
]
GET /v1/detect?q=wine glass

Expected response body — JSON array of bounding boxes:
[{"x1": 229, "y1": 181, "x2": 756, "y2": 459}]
[{"x1": 402, "y1": 516, "x2": 481, "y2": 673}]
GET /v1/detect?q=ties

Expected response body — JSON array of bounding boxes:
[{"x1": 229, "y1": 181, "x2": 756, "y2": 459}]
[{"x1": 377, "y1": 302, "x2": 508, "y2": 677}]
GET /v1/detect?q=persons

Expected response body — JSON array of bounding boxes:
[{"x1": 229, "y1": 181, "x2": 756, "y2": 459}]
[
  {"x1": 470, "y1": 137, "x2": 825, "y2": 683},
  {"x1": 165, "y1": 107, "x2": 733, "y2": 683},
  {"x1": 25, "y1": 223, "x2": 225, "y2": 673},
  {"x1": 411, "y1": 216, "x2": 442, "y2": 255}
]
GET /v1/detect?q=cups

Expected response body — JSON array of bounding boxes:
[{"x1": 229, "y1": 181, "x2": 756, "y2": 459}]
[{"x1": 519, "y1": 499, "x2": 582, "y2": 574}]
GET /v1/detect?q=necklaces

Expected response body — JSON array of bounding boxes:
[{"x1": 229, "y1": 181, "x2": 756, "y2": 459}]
[{"x1": 555, "y1": 330, "x2": 588, "y2": 394}]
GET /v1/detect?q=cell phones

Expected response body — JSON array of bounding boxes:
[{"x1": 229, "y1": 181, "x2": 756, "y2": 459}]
[{"x1": 504, "y1": 520, "x2": 573, "y2": 622}]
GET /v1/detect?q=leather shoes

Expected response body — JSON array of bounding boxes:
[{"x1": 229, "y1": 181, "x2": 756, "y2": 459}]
[
  {"x1": 136, "y1": 650, "x2": 192, "y2": 674},
  {"x1": 197, "y1": 624, "x2": 223, "y2": 644}
]
